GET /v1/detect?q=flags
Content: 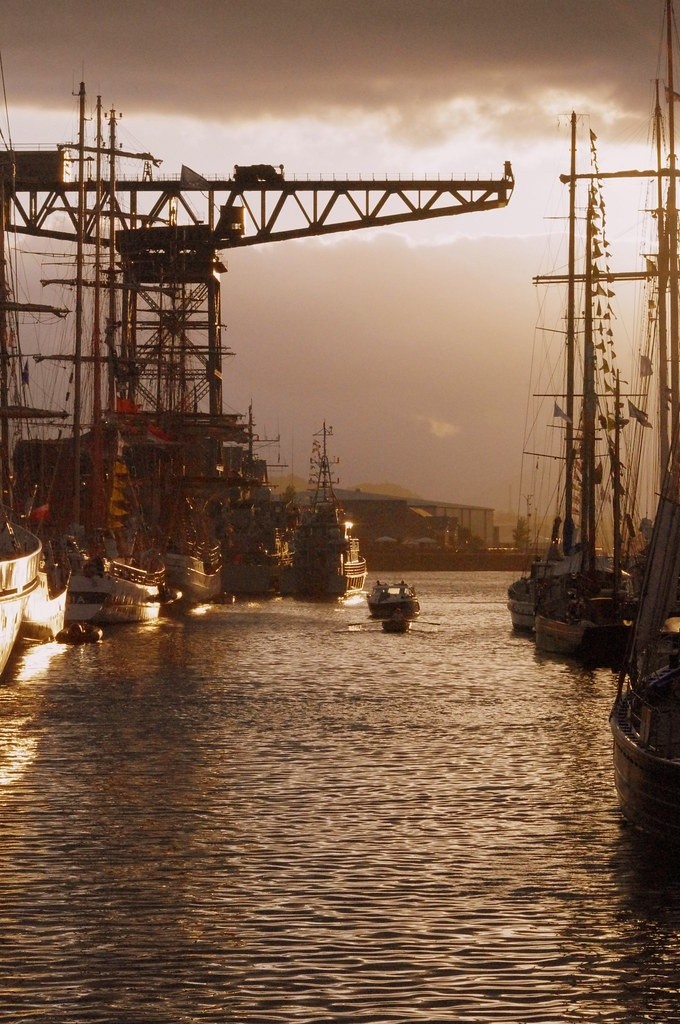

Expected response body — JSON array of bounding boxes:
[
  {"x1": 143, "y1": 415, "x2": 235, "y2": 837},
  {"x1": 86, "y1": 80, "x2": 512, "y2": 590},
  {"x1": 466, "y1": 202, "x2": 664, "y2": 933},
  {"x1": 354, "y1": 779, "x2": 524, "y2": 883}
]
[
  {"x1": 117, "y1": 398, "x2": 142, "y2": 413},
  {"x1": 32, "y1": 496, "x2": 49, "y2": 519},
  {"x1": 571, "y1": 128, "x2": 658, "y2": 537},
  {"x1": 665, "y1": 87, "x2": 680, "y2": 103},
  {"x1": 555, "y1": 403, "x2": 573, "y2": 425}
]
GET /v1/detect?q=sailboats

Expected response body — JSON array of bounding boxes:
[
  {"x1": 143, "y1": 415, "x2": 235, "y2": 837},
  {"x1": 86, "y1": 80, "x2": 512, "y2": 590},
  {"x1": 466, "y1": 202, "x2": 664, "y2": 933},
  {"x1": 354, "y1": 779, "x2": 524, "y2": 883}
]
[{"x1": 505, "y1": 0, "x2": 680, "y2": 858}]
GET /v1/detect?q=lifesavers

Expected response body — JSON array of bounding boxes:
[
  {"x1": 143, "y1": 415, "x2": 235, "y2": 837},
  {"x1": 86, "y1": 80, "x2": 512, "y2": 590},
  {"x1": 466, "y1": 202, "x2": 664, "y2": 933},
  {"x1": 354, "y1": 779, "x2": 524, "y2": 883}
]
[{"x1": 566, "y1": 601, "x2": 587, "y2": 622}]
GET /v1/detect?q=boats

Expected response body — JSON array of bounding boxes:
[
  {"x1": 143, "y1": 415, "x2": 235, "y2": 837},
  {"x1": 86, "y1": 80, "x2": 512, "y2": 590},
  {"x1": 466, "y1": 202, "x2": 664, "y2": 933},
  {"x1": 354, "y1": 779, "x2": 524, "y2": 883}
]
[
  {"x1": 222, "y1": 502, "x2": 367, "y2": 597},
  {"x1": 0, "y1": 81, "x2": 222, "y2": 679},
  {"x1": 366, "y1": 580, "x2": 420, "y2": 618},
  {"x1": 382, "y1": 620, "x2": 410, "y2": 632}
]
